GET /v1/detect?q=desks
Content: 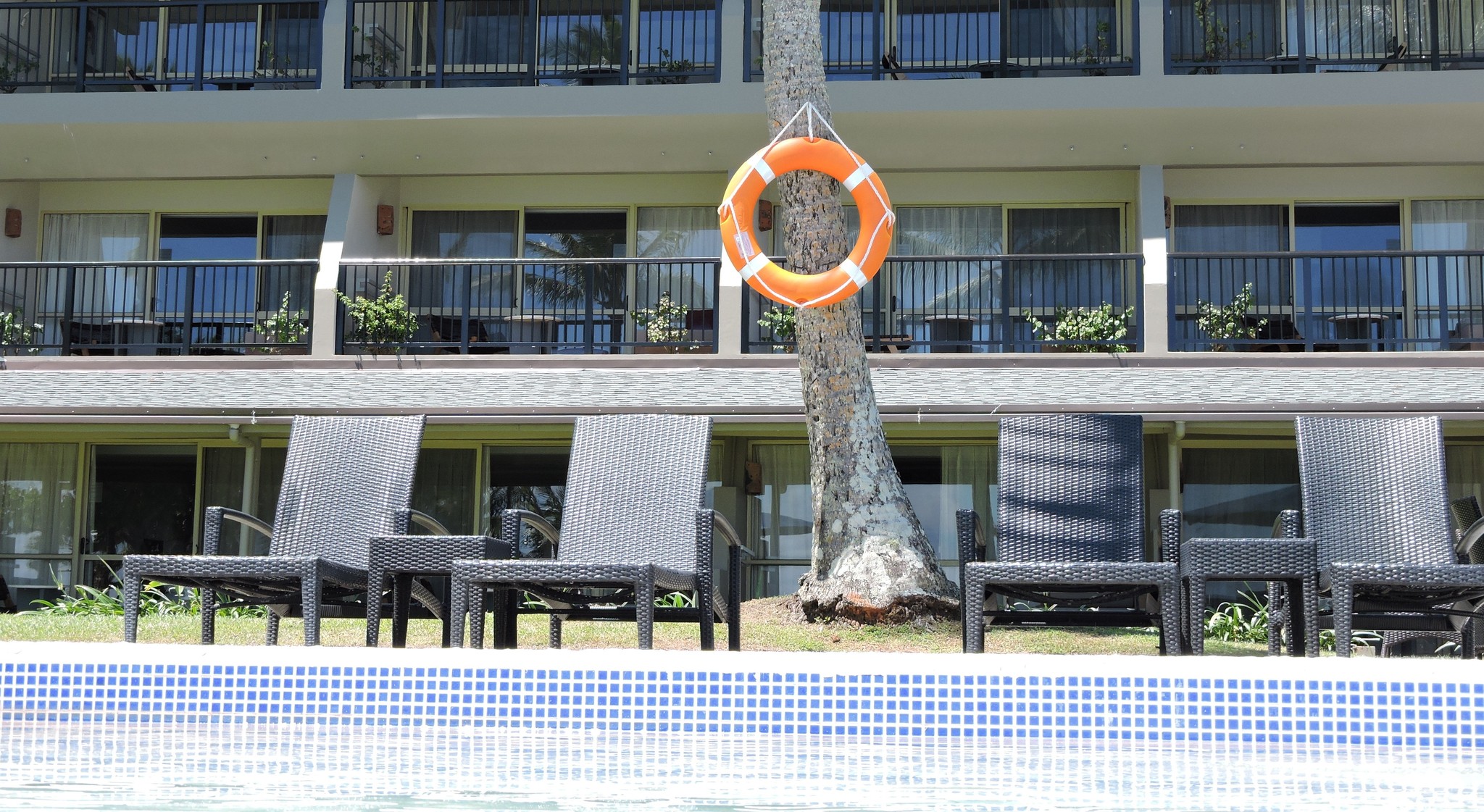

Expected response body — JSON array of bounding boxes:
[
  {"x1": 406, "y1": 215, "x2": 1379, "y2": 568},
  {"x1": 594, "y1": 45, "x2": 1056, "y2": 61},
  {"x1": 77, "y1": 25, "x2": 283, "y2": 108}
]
[
  {"x1": 1176, "y1": 539, "x2": 1320, "y2": 659},
  {"x1": 1328, "y1": 314, "x2": 1390, "y2": 352},
  {"x1": 210, "y1": 75, "x2": 254, "y2": 91},
  {"x1": 8, "y1": 584, "x2": 66, "y2": 614},
  {"x1": 109, "y1": 320, "x2": 164, "y2": 356},
  {"x1": 576, "y1": 67, "x2": 621, "y2": 86},
  {"x1": 924, "y1": 315, "x2": 977, "y2": 353},
  {"x1": 969, "y1": 62, "x2": 1025, "y2": 79},
  {"x1": 368, "y1": 534, "x2": 511, "y2": 648},
  {"x1": 504, "y1": 315, "x2": 561, "y2": 355},
  {"x1": 1265, "y1": 54, "x2": 1321, "y2": 74}
]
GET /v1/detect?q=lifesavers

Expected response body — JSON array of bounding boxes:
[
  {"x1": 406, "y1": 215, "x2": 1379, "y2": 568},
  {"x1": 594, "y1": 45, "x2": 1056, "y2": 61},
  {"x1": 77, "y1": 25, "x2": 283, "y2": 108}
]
[{"x1": 715, "y1": 134, "x2": 897, "y2": 313}]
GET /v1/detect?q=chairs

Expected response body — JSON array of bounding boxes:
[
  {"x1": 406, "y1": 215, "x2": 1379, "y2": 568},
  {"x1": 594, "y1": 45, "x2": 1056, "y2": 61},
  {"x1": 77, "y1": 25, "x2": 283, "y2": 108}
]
[
  {"x1": 957, "y1": 415, "x2": 1484, "y2": 657},
  {"x1": 60, "y1": 319, "x2": 114, "y2": 356},
  {"x1": 126, "y1": 67, "x2": 157, "y2": 92},
  {"x1": 427, "y1": 314, "x2": 510, "y2": 355},
  {"x1": 882, "y1": 51, "x2": 910, "y2": 80},
  {"x1": 1320, "y1": 36, "x2": 1407, "y2": 73},
  {"x1": 1241, "y1": 317, "x2": 1337, "y2": 352},
  {"x1": 863, "y1": 334, "x2": 912, "y2": 354},
  {"x1": 123, "y1": 415, "x2": 754, "y2": 649}
]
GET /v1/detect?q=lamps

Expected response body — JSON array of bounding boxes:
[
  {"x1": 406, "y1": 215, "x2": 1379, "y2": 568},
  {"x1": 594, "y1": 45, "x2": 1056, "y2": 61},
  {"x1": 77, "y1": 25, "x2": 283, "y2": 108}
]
[
  {"x1": 746, "y1": 460, "x2": 762, "y2": 495},
  {"x1": 5, "y1": 208, "x2": 22, "y2": 238},
  {"x1": 378, "y1": 205, "x2": 395, "y2": 236}
]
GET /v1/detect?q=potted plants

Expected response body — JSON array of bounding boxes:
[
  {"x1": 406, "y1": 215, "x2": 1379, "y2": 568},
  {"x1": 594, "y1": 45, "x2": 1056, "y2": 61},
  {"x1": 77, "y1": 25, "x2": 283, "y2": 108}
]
[{"x1": 255, "y1": 270, "x2": 419, "y2": 356}]
